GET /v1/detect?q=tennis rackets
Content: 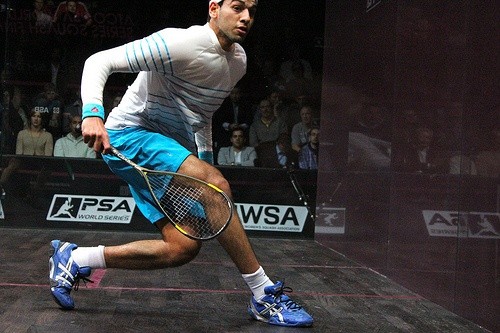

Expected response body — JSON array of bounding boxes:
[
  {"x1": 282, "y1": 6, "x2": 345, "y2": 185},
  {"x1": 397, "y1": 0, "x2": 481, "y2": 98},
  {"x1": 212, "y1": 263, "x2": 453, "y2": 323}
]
[{"x1": 108, "y1": 146, "x2": 233, "y2": 242}]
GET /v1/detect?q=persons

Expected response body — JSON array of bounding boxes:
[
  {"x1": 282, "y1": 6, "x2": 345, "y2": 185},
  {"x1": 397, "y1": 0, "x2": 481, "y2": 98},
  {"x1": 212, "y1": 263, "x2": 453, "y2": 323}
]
[
  {"x1": 0, "y1": 0, "x2": 323, "y2": 168},
  {"x1": 331, "y1": 85, "x2": 500, "y2": 178},
  {"x1": 47, "y1": 0, "x2": 313, "y2": 327}
]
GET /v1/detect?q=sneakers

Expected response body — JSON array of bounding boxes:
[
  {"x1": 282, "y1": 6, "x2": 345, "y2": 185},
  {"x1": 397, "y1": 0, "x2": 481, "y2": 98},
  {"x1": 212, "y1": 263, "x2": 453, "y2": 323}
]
[
  {"x1": 46, "y1": 239, "x2": 94, "y2": 310},
  {"x1": 248, "y1": 278, "x2": 314, "y2": 327}
]
